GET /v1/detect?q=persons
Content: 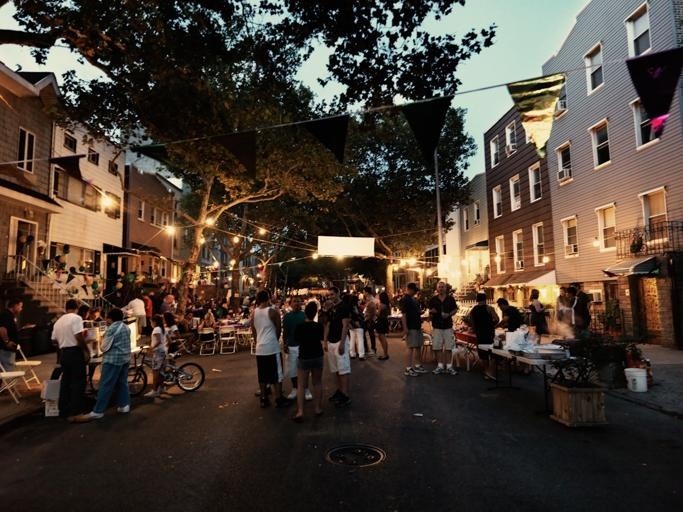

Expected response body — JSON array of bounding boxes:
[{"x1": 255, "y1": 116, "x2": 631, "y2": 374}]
[
  {"x1": 394, "y1": 279, "x2": 592, "y2": 376},
  {"x1": 47, "y1": 274, "x2": 199, "y2": 419},
  {"x1": 204, "y1": 285, "x2": 393, "y2": 420},
  {"x1": 0, "y1": 298, "x2": 30, "y2": 394}
]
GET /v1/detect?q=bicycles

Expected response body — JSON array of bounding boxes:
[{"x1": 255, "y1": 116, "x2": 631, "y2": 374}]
[{"x1": 124, "y1": 346, "x2": 204, "y2": 396}]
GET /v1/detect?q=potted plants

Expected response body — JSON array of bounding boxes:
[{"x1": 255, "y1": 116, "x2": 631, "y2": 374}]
[{"x1": 541, "y1": 318, "x2": 619, "y2": 432}]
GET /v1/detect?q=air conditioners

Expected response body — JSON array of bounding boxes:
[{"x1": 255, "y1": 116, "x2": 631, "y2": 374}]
[
  {"x1": 564, "y1": 242, "x2": 578, "y2": 257},
  {"x1": 552, "y1": 98, "x2": 566, "y2": 116},
  {"x1": 556, "y1": 168, "x2": 570, "y2": 183},
  {"x1": 504, "y1": 142, "x2": 515, "y2": 155},
  {"x1": 514, "y1": 259, "x2": 522, "y2": 272}
]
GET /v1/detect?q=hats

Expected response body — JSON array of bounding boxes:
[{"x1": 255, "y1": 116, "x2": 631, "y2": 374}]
[
  {"x1": 407, "y1": 283, "x2": 419, "y2": 292},
  {"x1": 420, "y1": 308, "x2": 431, "y2": 318},
  {"x1": 476, "y1": 293, "x2": 487, "y2": 302}
]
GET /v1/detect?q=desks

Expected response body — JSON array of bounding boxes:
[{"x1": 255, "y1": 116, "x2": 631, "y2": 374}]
[
  {"x1": 451, "y1": 330, "x2": 477, "y2": 372},
  {"x1": 477, "y1": 344, "x2": 576, "y2": 418}
]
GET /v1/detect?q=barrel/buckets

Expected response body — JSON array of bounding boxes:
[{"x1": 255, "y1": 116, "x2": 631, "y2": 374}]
[{"x1": 624, "y1": 367, "x2": 647, "y2": 394}]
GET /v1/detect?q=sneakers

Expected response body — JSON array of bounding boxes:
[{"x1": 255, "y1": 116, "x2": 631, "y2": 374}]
[
  {"x1": 67, "y1": 414, "x2": 89, "y2": 422},
  {"x1": 157, "y1": 386, "x2": 166, "y2": 392},
  {"x1": 144, "y1": 389, "x2": 160, "y2": 397},
  {"x1": 83, "y1": 411, "x2": 104, "y2": 419},
  {"x1": 403, "y1": 367, "x2": 418, "y2": 376},
  {"x1": 415, "y1": 363, "x2": 429, "y2": 373},
  {"x1": 350, "y1": 348, "x2": 392, "y2": 361},
  {"x1": 482, "y1": 371, "x2": 497, "y2": 384},
  {"x1": 445, "y1": 364, "x2": 458, "y2": 376},
  {"x1": 117, "y1": 404, "x2": 130, "y2": 412},
  {"x1": 254, "y1": 386, "x2": 352, "y2": 409},
  {"x1": 432, "y1": 362, "x2": 445, "y2": 375}
]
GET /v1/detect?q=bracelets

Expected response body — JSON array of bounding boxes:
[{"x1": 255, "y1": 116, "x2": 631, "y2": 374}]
[{"x1": 3, "y1": 338, "x2": 10, "y2": 345}]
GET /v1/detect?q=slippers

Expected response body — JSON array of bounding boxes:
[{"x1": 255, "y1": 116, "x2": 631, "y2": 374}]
[
  {"x1": 313, "y1": 410, "x2": 327, "y2": 416},
  {"x1": 289, "y1": 414, "x2": 305, "y2": 422}
]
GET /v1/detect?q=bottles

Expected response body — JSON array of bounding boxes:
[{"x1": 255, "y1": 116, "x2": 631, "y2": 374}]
[{"x1": 526, "y1": 326, "x2": 536, "y2": 345}]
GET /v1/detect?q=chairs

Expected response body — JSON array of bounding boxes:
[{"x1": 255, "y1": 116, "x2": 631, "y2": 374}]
[
  {"x1": 0, "y1": 360, "x2": 26, "y2": 405},
  {"x1": 13, "y1": 344, "x2": 42, "y2": 391},
  {"x1": 421, "y1": 330, "x2": 436, "y2": 362},
  {"x1": 175, "y1": 304, "x2": 285, "y2": 356}
]
